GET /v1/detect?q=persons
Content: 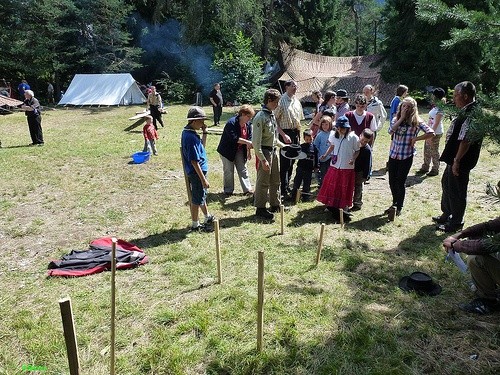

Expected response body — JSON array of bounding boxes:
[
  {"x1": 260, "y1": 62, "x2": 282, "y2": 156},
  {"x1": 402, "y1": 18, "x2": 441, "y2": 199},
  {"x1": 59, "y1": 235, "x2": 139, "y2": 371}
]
[
  {"x1": 181, "y1": 106, "x2": 225, "y2": 232},
  {"x1": 312, "y1": 84, "x2": 387, "y2": 191},
  {"x1": 209, "y1": 83, "x2": 224, "y2": 126},
  {"x1": 352, "y1": 128, "x2": 373, "y2": 210},
  {"x1": 148, "y1": 86, "x2": 164, "y2": 131},
  {"x1": 431, "y1": 81, "x2": 484, "y2": 232},
  {"x1": 18, "y1": 80, "x2": 30, "y2": 101},
  {"x1": 252, "y1": 89, "x2": 292, "y2": 220},
  {"x1": 317, "y1": 116, "x2": 361, "y2": 209},
  {"x1": 143, "y1": 115, "x2": 158, "y2": 156},
  {"x1": 292, "y1": 129, "x2": 319, "y2": 204},
  {"x1": 217, "y1": 104, "x2": 254, "y2": 197},
  {"x1": 22, "y1": 90, "x2": 44, "y2": 146},
  {"x1": 46, "y1": 81, "x2": 56, "y2": 108},
  {"x1": 274, "y1": 80, "x2": 304, "y2": 200},
  {"x1": 385, "y1": 85, "x2": 445, "y2": 216},
  {"x1": 443, "y1": 181, "x2": 500, "y2": 317}
]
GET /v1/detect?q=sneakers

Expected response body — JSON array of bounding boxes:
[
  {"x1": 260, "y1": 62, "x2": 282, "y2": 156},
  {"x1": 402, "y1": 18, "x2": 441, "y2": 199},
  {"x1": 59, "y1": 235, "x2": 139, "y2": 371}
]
[{"x1": 459, "y1": 298, "x2": 500, "y2": 313}]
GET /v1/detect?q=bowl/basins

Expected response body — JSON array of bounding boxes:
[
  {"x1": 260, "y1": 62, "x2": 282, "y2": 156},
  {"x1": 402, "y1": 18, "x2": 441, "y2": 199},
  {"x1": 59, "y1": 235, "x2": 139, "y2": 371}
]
[{"x1": 131, "y1": 152, "x2": 151, "y2": 163}]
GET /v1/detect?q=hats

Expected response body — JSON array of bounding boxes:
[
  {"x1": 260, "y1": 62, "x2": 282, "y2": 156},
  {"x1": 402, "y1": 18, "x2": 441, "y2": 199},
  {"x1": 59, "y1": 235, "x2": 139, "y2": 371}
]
[
  {"x1": 399, "y1": 272, "x2": 442, "y2": 297},
  {"x1": 337, "y1": 89, "x2": 349, "y2": 98},
  {"x1": 431, "y1": 88, "x2": 445, "y2": 99},
  {"x1": 334, "y1": 116, "x2": 351, "y2": 128},
  {"x1": 184, "y1": 106, "x2": 207, "y2": 120},
  {"x1": 281, "y1": 143, "x2": 307, "y2": 159}
]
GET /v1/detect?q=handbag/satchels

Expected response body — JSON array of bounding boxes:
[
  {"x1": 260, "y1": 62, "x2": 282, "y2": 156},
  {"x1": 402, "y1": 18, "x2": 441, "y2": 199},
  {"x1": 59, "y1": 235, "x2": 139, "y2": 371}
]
[
  {"x1": 19, "y1": 86, "x2": 25, "y2": 92},
  {"x1": 331, "y1": 154, "x2": 337, "y2": 164}
]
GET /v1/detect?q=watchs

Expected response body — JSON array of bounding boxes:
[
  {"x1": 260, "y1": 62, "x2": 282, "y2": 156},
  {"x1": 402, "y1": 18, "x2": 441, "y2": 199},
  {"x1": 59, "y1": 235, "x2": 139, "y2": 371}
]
[{"x1": 450, "y1": 240, "x2": 457, "y2": 248}]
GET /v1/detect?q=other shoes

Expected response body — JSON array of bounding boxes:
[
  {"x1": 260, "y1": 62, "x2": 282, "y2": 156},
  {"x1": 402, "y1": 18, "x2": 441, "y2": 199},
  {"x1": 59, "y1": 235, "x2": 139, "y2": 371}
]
[
  {"x1": 256, "y1": 209, "x2": 273, "y2": 218},
  {"x1": 208, "y1": 216, "x2": 225, "y2": 228},
  {"x1": 244, "y1": 191, "x2": 254, "y2": 195},
  {"x1": 38, "y1": 143, "x2": 44, "y2": 146},
  {"x1": 416, "y1": 168, "x2": 429, "y2": 173},
  {"x1": 269, "y1": 205, "x2": 290, "y2": 212},
  {"x1": 225, "y1": 192, "x2": 232, "y2": 196},
  {"x1": 29, "y1": 143, "x2": 39, "y2": 145},
  {"x1": 192, "y1": 224, "x2": 213, "y2": 232},
  {"x1": 436, "y1": 222, "x2": 464, "y2": 233},
  {"x1": 432, "y1": 216, "x2": 451, "y2": 223},
  {"x1": 428, "y1": 170, "x2": 439, "y2": 175}
]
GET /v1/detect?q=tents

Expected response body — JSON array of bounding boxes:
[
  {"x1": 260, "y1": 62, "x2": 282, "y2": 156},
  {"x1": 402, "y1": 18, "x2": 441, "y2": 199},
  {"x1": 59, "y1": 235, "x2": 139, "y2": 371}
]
[{"x1": 57, "y1": 74, "x2": 148, "y2": 108}]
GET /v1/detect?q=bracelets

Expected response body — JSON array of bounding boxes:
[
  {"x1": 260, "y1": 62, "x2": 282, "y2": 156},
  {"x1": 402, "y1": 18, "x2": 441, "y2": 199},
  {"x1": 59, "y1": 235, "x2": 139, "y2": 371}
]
[
  {"x1": 454, "y1": 158, "x2": 459, "y2": 163},
  {"x1": 203, "y1": 132, "x2": 207, "y2": 134}
]
